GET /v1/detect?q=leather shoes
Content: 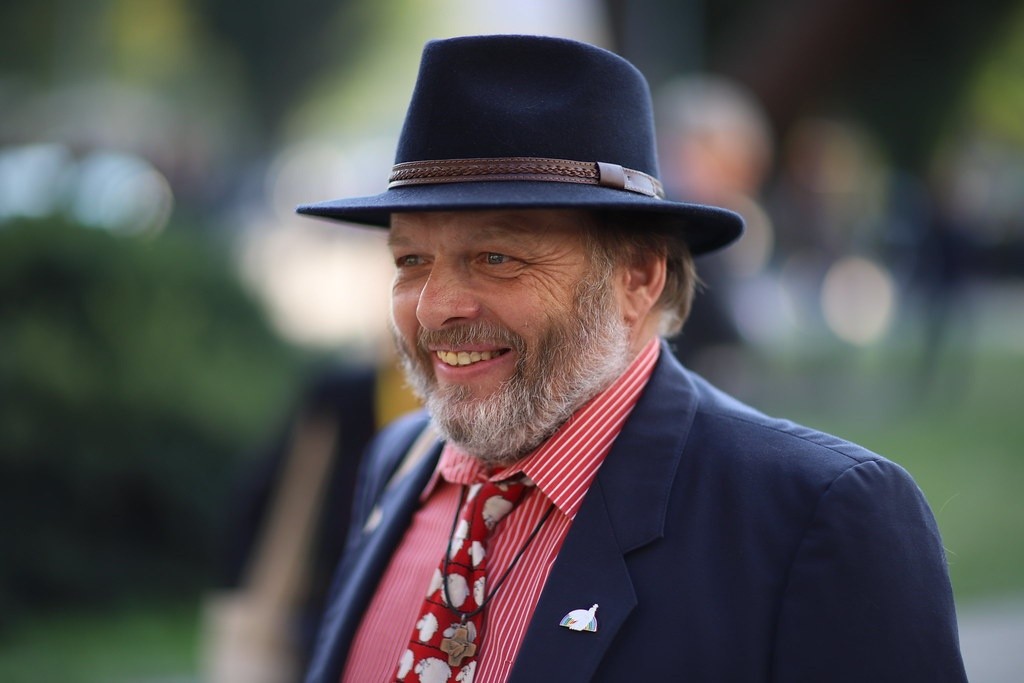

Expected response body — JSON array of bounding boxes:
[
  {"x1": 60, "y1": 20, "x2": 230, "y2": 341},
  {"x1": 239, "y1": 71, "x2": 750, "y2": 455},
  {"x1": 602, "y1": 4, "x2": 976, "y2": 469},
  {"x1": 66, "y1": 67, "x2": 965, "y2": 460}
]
[{"x1": 391, "y1": 471, "x2": 535, "y2": 683}]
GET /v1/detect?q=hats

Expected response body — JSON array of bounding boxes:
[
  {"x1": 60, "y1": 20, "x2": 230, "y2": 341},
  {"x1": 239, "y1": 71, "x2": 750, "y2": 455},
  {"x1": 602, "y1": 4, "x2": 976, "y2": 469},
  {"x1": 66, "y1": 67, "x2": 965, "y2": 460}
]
[{"x1": 296, "y1": 34, "x2": 744, "y2": 264}]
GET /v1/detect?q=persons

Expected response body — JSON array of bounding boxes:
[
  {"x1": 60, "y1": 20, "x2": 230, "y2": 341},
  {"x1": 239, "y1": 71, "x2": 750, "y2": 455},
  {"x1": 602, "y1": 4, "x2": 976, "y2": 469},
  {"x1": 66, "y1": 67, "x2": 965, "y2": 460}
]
[{"x1": 295, "y1": 34, "x2": 969, "y2": 683}]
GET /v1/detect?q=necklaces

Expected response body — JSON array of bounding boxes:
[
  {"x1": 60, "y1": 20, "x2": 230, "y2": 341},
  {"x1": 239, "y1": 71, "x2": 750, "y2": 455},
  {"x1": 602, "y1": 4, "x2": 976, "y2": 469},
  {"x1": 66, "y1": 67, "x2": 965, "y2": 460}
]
[{"x1": 440, "y1": 484, "x2": 555, "y2": 667}]
